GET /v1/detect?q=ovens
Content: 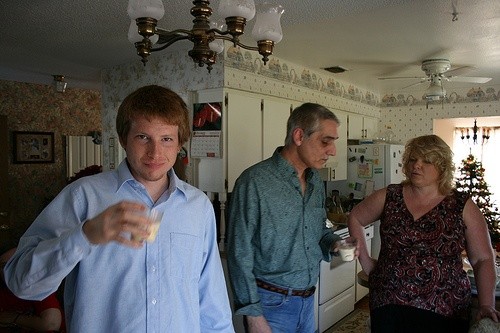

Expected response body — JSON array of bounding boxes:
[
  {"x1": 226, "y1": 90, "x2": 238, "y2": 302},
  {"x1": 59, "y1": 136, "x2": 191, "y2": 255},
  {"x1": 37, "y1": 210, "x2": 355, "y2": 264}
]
[{"x1": 319, "y1": 239, "x2": 354, "y2": 333}]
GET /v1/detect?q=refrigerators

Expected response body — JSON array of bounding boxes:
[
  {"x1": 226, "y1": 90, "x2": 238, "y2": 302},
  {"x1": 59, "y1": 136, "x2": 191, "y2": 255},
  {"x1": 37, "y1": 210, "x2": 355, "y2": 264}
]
[{"x1": 344, "y1": 141, "x2": 409, "y2": 265}]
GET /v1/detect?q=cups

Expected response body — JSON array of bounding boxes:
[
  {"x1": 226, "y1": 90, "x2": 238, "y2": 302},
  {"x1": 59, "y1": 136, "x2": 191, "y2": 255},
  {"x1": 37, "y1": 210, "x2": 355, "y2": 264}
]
[
  {"x1": 338, "y1": 245, "x2": 356, "y2": 261},
  {"x1": 132, "y1": 208, "x2": 165, "y2": 242}
]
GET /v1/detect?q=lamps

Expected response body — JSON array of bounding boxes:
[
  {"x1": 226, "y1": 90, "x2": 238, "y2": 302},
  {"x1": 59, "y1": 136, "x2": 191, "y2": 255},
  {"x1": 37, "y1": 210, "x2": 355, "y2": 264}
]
[
  {"x1": 421, "y1": 82, "x2": 447, "y2": 110},
  {"x1": 53, "y1": 75, "x2": 67, "y2": 93},
  {"x1": 127, "y1": 0, "x2": 285, "y2": 73}
]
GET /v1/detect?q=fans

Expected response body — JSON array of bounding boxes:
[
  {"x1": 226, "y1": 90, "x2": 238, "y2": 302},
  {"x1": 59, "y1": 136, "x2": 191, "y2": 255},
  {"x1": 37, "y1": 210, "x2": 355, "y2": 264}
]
[{"x1": 377, "y1": 60, "x2": 492, "y2": 89}]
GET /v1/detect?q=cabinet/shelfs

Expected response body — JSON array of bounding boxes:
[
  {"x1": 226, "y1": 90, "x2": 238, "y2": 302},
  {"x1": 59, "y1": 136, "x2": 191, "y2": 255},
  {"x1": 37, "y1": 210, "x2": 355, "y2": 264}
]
[
  {"x1": 318, "y1": 256, "x2": 356, "y2": 333},
  {"x1": 197, "y1": 90, "x2": 378, "y2": 192}
]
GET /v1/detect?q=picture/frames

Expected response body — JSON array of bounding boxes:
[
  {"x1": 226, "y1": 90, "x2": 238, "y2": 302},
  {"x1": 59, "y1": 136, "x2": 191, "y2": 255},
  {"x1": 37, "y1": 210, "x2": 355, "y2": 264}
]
[{"x1": 13, "y1": 131, "x2": 54, "y2": 163}]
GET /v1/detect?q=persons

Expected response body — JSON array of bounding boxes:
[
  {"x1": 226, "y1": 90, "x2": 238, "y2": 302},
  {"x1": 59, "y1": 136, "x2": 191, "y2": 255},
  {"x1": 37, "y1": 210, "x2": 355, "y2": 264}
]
[
  {"x1": 224, "y1": 103, "x2": 360, "y2": 333},
  {"x1": 4, "y1": 85, "x2": 236, "y2": 333},
  {"x1": 348, "y1": 135, "x2": 500, "y2": 333}
]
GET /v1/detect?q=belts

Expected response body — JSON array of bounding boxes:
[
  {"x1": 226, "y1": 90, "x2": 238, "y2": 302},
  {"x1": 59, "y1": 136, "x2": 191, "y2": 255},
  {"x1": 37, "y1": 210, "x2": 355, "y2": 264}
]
[{"x1": 256, "y1": 278, "x2": 317, "y2": 298}]
